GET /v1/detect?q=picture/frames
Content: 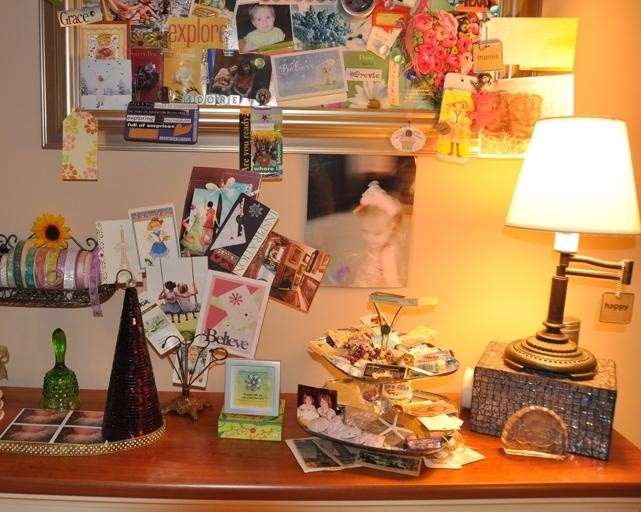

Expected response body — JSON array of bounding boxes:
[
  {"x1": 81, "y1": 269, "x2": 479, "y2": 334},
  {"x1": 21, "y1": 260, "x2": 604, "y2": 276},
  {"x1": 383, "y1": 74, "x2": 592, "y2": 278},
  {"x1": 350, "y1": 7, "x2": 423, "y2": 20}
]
[
  {"x1": 226, "y1": 358, "x2": 280, "y2": 414},
  {"x1": 40, "y1": 0, "x2": 545, "y2": 154}
]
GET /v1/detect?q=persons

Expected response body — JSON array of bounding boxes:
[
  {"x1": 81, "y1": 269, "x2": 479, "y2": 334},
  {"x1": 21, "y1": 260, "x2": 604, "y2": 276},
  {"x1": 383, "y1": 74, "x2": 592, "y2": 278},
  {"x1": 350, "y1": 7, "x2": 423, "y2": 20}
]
[
  {"x1": 167, "y1": 282, "x2": 199, "y2": 320},
  {"x1": 317, "y1": 393, "x2": 337, "y2": 420},
  {"x1": 178, "y1": 346, "x2": 205, "y2": 385},
  {"x1": 298, "y1": 392, "x2": 319, "y2": 420},
  {"x1": 159, "y1": 280, "x2": 182, "y2": 323},
  {"x1": 352, "y1": 180, "x2": 406, "y2": 287},
  {"x1": 239, "y1": 3, "x2": 286, "y2": 53},
  {"x1": 233, "y1": 61, "x2": 255, "y2": 97},
  {"x1": 213, "y1": 63, "x2": 239, "y2": 95}
]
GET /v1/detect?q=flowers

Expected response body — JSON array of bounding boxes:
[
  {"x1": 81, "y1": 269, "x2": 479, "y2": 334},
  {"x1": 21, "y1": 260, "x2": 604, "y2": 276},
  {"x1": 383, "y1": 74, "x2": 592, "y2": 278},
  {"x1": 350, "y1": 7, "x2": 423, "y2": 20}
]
[
  {"x1": 32, "y1": 213, "x2": 73, "y2": 250},
  {"x1": 401, "y1": 1, "x2": 482, "y2": 104}
]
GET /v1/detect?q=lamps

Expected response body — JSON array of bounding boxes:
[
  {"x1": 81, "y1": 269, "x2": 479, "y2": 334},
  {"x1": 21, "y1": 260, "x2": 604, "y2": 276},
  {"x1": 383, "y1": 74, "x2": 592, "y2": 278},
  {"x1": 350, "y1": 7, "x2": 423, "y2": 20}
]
[{"x1": 503, "y1": 117, "x2": 641, "y2": 378}]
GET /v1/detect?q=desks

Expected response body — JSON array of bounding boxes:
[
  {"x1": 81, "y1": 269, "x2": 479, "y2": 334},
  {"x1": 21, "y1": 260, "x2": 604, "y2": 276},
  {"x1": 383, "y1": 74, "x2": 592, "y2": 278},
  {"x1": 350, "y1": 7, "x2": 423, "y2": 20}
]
[{"x1": 2, "y1": 386, "x2": 641, "y2": 510}]
